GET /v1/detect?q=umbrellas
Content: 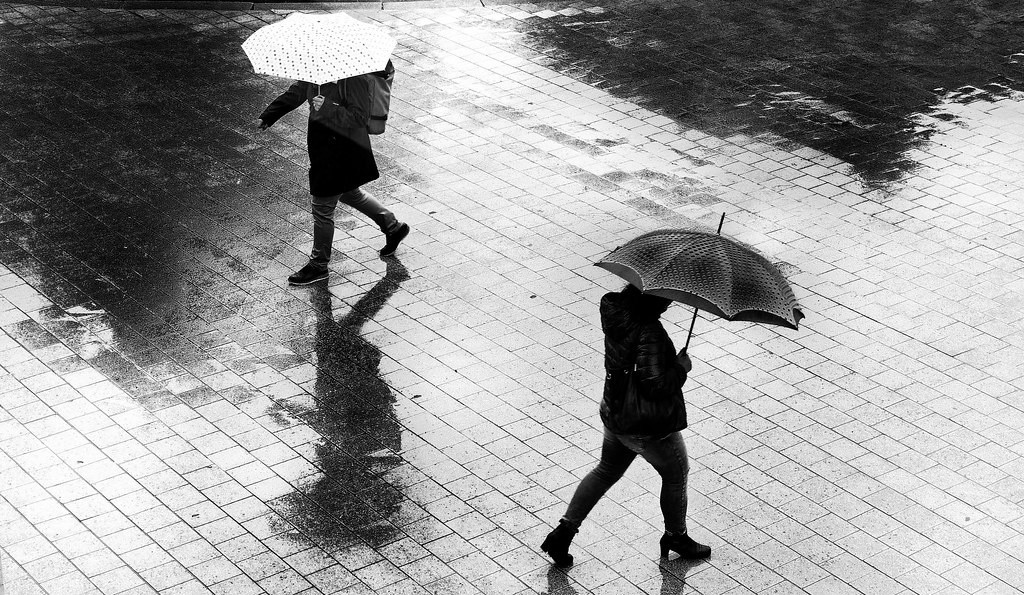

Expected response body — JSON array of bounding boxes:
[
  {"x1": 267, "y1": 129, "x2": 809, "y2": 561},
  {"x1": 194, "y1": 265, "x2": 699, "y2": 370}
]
[
  {"x1": 240, "y1": 12, "x2": 397, "y2": 97},
  {"x1": 591, "y1": 209, "x2": 805, "y2": 356}
]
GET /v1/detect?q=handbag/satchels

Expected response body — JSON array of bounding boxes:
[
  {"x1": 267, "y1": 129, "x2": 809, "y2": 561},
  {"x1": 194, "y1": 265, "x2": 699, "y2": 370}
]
[{"x1": 620, "y1": 383, "x2": 687, "y2": 438}]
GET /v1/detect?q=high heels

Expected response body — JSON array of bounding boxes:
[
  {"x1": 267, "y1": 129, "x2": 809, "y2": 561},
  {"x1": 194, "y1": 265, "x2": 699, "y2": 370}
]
[
  {"x1": 541, "y1": 518, "x2": 579, "y2": 566},
  {"x1": 659, "y1": 528, "x2": 711, "y2": 559}
]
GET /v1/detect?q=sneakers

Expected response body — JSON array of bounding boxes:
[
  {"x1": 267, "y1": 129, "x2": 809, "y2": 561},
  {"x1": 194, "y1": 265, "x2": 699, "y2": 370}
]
[
  {"x1": 379, "y1": 222, "x2": 410, "y2": 256},
  {"x1": 288, "y1": 263, "x2": 330, "y2": 286}
]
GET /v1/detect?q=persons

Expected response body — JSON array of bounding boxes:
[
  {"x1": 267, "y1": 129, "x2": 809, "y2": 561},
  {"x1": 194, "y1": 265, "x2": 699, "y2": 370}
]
[
  {"x1": 252, "y1": 76, "x2": 409, "y2": 286},
  {"x1": 538, "y1": 283, "x2": 713, "y2": 569}
]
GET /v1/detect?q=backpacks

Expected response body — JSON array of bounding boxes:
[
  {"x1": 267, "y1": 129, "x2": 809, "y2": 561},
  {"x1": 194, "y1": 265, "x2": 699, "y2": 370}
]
[{"x1": 339, "y1": 59, "x2": 395, "y2": 135}]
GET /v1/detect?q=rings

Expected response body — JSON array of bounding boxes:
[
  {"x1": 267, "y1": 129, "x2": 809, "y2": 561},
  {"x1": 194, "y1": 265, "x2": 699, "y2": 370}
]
[{"x1": 314, "y1": 104, "x2": 316, "y2": 107}]
